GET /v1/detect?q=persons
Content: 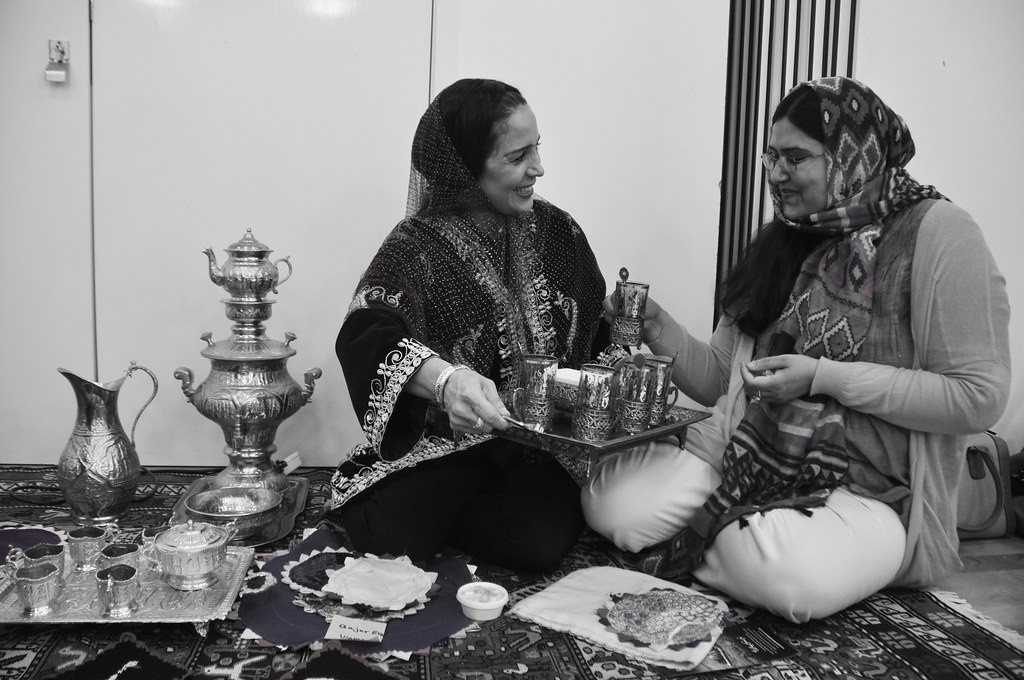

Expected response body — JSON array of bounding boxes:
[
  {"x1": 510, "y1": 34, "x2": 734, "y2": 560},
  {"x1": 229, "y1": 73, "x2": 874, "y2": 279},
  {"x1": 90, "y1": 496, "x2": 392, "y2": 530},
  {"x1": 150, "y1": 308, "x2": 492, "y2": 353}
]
[
  {"x1": 336, "y1": 77, "x2": 634, "y2": 570},
  {"x1": 583, "y1": 77, "x2": 1014, "y2": 621}
]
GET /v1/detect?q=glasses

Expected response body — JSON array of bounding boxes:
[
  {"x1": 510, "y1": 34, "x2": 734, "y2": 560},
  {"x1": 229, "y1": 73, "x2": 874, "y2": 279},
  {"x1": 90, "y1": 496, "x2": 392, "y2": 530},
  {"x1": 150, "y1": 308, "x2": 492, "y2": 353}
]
[{"x1": 760, "y1": 153, "x2": 824, "y2": 172}]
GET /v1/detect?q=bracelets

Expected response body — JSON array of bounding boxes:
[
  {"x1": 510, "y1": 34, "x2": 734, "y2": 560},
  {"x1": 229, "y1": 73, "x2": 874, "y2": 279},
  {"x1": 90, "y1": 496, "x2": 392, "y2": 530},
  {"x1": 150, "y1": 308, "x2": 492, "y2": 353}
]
[{"x1": 436, "y1": 365, "x2": 473, "y2": 413}]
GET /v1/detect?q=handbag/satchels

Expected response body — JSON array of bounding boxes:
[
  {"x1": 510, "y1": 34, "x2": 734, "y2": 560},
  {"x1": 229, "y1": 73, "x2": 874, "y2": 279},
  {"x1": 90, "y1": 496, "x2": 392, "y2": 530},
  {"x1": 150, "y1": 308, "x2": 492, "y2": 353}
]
[{"x1": 957, "y1": 429, "x2": 1015, "y2": 540}]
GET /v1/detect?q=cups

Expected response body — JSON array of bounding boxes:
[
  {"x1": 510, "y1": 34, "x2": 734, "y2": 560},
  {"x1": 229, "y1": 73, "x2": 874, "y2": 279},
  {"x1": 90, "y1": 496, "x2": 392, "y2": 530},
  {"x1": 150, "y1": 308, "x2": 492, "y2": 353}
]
[
  {"x1": 101, "y1": 542, "x2": 139, "y2": 579},
  {"x1": 621, "y1": 362, "x2": 656, "y2": 433},
  {"x1": 23, "y1": 545, "x2": 65, "y2": 577},
  {"x1": 94, "y1": 564, "x2": 137, "y2": 617},
  {"x1": 511, "y1": 357, "x2": 556, "y2": 429},
  {"x1": 611, "y1": 278, "x2": 648, "y2": 345},
  {"x1": 68, "y1": 527, "x2": 103, "y2": 570},
  {"x1": 572, "y1": 362, "x2": 623, "y2": 439},
  {"x1": 646, "y1": 360, "x2": 678, "y2": 426},
  {"x1": 13, "y1": 564, "x2": 58, "y2": 618}
]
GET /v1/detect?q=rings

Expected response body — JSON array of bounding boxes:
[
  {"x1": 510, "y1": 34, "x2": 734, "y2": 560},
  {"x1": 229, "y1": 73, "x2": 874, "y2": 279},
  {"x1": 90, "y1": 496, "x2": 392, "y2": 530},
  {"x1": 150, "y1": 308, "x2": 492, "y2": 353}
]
[
  {"x1": 751, "y1": 390, "x2": 764, "y2": 403},
  {"x1": 472, "y1": 417, "x2": 487, "y2": 429}
]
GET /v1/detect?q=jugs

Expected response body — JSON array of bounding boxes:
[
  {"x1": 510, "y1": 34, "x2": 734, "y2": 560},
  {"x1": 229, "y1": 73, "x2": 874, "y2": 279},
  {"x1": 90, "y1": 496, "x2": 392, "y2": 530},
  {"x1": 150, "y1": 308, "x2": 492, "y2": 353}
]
[
  {"x1": 144, "y1": 519, "x2": 240, "y2": 592},
  {"x1": 56, "y1": 362, "x2": 160, "y2": 521}
]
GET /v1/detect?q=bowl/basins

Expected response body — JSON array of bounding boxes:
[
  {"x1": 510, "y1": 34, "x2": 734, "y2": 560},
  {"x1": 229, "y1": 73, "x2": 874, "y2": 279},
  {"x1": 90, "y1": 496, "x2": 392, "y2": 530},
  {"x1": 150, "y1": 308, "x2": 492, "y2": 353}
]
[
  {"x1": 184, "y1": 487, "x2": 283, "y2": 541},
  {"x1": 457, "y1": 581, "x2": 509, "y2": 620}
]
